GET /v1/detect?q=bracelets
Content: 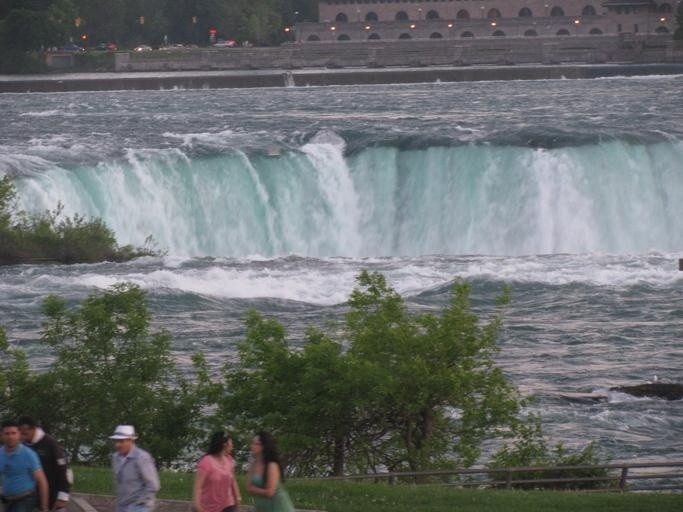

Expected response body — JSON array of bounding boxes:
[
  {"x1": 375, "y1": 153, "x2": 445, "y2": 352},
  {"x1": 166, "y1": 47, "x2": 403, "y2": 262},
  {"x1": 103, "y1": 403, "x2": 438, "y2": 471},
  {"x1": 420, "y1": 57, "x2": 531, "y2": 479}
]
[
  {"x1": 40, "y1": 503, "x2": 48, "y2": 509},
  {"x1": 235, "y1": 497, "x2": 241, "y2": 501}
]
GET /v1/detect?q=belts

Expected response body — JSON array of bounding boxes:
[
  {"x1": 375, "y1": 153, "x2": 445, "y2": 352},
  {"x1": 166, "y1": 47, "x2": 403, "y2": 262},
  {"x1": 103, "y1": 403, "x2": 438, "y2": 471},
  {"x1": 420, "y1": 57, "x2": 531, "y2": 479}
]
[{"x1": 3, "y1": 487, "x2": 37, "y2": 502}]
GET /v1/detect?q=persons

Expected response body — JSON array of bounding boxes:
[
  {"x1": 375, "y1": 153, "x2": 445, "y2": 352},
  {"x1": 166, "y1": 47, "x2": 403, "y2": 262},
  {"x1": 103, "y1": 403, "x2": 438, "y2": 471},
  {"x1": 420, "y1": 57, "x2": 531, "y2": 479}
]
[
  {"x1": 106, "y1": 425, "x2": 161, "y2": 511},
  {"x1": 188, "y1": 429, "x2": 243, "y2": 512},
  {"x1": 244, "y1": 431, "x2": 295, "y2": 512},
  {"x1": 0, "y1": 420, "x2": 50, "y2": 511},
  {"x1": 18, "y1": 415, "x2": 74, "y2": 512}
]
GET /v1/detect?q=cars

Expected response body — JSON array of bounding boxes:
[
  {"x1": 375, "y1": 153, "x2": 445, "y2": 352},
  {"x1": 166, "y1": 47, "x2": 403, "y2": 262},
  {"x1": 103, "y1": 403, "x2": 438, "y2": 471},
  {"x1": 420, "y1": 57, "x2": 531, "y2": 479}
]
[{"x1": 59, "y1": 40, "x2": 242, "y2": 52}]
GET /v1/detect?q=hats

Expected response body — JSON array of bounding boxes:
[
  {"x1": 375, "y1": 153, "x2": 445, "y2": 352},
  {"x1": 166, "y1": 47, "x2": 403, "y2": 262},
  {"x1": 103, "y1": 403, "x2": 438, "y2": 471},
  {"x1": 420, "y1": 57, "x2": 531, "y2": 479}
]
[{"x1": 108, "y1": 424, "x2": 139, "y2": 441}]
